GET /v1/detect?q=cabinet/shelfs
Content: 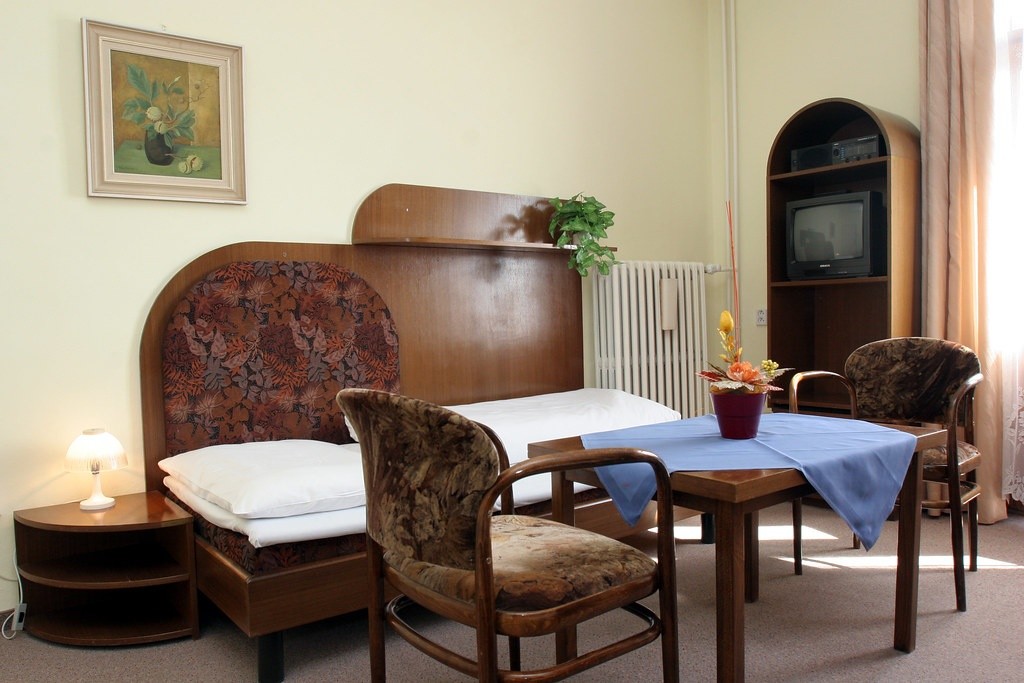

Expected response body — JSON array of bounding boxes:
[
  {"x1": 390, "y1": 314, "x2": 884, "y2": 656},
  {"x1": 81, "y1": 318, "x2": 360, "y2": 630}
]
[
  {"x1": 13, "y1": 489, "x2": 200, "y2": 648},
  {"x1": 765, "y1": 98, "x2": 923, "y2": 522}
]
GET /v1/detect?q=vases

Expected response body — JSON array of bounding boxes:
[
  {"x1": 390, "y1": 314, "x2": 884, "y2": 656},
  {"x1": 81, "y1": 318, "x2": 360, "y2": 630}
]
[{"x1": 711, "y1": 392, "x2": 765, "y2": 439}]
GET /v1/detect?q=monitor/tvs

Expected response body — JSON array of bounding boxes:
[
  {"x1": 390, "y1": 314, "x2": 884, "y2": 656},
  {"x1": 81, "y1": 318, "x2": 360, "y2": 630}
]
[{"x1": 785, "y1": 191, "x2": 886, "y2": 279}]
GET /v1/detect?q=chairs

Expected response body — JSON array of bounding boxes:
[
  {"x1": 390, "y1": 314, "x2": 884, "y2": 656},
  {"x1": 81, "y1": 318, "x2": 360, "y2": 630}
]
[
  {"x1": 337, "y1": 388, "x2": 680, "y2": 683},
  {"x1": 788, "y1": 338, "x2": 984, "y2": 612}
]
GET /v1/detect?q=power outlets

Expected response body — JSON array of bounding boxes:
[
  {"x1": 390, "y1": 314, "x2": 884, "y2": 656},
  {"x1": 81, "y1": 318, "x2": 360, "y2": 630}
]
[{"x1": 756, "y1": 310, "x2": 767, "y2": 326}]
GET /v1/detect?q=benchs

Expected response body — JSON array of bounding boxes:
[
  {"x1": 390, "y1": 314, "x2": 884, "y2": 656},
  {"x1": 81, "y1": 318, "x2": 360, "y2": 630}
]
[{"x1": 140, "y1": 240, "x2": 715, "y2": 682}]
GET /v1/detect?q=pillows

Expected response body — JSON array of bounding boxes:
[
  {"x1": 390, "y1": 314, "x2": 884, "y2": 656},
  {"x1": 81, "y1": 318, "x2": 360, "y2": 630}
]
[{"x1": 156, "y1": 439, "x2": 366, "y2": 519}]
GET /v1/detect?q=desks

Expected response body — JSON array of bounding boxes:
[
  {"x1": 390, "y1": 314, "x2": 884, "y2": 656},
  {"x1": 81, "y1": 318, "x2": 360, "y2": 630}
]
[{"x1": 529, "y1": 407, "x2": 948, "y2": 683}]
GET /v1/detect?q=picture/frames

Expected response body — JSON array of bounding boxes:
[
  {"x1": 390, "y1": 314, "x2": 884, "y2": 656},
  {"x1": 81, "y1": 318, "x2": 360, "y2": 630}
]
[{"x1": 80, "y1": 19, "x2": 248, "y2": 204}]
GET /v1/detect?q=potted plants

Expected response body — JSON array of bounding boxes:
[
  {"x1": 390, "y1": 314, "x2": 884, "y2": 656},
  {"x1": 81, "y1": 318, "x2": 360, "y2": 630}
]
[{"x1": 548, "y1": 192, "x2": 623, "y2": 278}]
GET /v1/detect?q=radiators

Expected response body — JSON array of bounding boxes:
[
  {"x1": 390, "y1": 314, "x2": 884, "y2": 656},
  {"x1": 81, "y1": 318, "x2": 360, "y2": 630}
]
[{"x1": 590, "y1": 262, "x2": 720, "y2": 421}]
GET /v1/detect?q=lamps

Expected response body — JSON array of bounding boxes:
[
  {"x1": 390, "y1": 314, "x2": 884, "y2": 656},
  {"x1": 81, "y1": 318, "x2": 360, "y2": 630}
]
[{"x1": 63, "y1": 428, "x2": 129, "y2": 511}]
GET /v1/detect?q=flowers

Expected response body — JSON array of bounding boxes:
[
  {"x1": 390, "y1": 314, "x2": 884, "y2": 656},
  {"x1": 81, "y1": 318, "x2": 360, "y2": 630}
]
[{"x1": 694, "y1": 311, "x2": 794, "y2": 394}]
